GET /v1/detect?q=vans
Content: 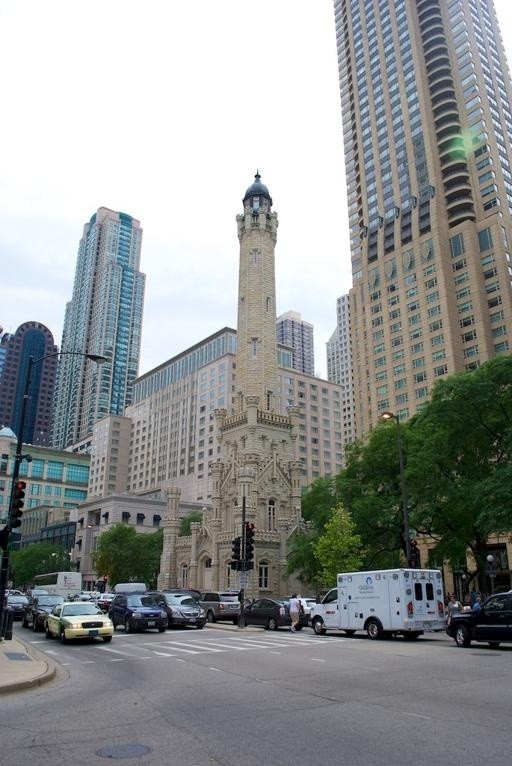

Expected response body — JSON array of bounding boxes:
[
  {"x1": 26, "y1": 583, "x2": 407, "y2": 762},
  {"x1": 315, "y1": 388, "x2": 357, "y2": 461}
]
[{"x1": 148, "y1": 588, "x2": 207, "y2": 629}]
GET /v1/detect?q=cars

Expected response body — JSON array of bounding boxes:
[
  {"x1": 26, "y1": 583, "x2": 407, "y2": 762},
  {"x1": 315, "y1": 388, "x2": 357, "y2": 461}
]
[
  {"x1": 287, "y1": 598, "x2": 316, "y2": 621},
  {"x1": 4, "y1": 589, "x2": 33, "y2": 621},
  {"x1": 44, "y1": 602, "x2": 114, "y2": 644},
  {"x1": 109, "y1": 595, "x2": 168, "y2": 633},
  {"x1": 238, "y1": 598, "x2": 288, "y2": 630},
  {"x1": 70, "y1": 591, "x2": 101, "y2": 602},
  {"x1": 22, "y1": 594, "x2": 66, "y2": 632},
  {"x1": 87, "y1": 594, "x2": 114, "y2": 610},
  {"x1": 26, "y1": 590, "x2": 49, "y2": 602},
  {"x1": 446, "y1": 591, "x2": 512, "y2": 649}
]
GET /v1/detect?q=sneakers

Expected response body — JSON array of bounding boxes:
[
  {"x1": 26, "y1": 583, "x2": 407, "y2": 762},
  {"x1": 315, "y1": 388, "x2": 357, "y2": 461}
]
[{"x1": 290, "y1": 626, "x2": 295, "y2": 633}]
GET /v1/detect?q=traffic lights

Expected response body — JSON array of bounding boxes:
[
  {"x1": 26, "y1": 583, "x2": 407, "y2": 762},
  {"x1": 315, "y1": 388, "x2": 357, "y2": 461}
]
[
  {"x1": 232, "y1": 537, "x2": 243, "y2": 560},
  {"x1": 7, "y1": 477, "x2": 26, "y2": 530},
  {"x1": 244, "y1": 523, "x2": 254, "y2": 560},
  {"x1": 7, "y1": 532, "x2": 21, "y2": 553},
  {"x1": 407, "y1": 538, "x2": 418, "y2": 568}
]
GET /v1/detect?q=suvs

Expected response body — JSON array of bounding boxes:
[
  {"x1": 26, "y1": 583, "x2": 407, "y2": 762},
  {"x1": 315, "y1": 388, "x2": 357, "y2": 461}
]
[{"x1": 199, "y1": 592, "x2": 240, "y2": 624}]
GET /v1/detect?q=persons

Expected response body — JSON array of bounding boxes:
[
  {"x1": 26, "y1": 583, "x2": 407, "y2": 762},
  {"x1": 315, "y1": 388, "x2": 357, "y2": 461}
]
[
  {"x1": 286, "y1": 592, "x2": 303, "y2": 633},
  {"x1": 446, "y1": 595, "x2": 464, "y2": 626},
  {"x1": 472, "y1": 596, "x2": 482, "y2": 613},
  {"x1": 461, "y1": 601, "x2": 472, "y2": 613}
]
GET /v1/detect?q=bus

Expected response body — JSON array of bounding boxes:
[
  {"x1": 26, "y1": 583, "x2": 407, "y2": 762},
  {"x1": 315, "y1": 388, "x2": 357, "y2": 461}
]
[{"x1": 30, "y1": 572, "x2": 82, "y2": 602}]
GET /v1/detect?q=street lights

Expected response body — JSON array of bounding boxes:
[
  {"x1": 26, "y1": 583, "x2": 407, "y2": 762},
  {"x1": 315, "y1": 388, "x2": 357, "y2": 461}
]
[
  {"x1": 381, "y1": 411, "x2": 411, "y2": 561},
  {"x1": 487, "y1": 553, "x2": 497, "y2": 594},
  {"x1": 87, "y1": 525, "x2": 108, "y2": 531},
  {"x1": 1, "y1": 352, "x2": 109, "y2": 631}
]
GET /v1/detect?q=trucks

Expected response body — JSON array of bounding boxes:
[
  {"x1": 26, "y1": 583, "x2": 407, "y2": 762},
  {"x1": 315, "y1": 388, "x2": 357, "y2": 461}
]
[
  {"x1": 113, "y1": 583, "x2": 146, "y2": 592},
  {"x1": 310, "y1": 569, "x2": 447, "y2": 640}
]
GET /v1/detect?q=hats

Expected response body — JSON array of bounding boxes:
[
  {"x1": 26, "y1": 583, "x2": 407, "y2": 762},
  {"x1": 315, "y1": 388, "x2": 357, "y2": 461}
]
[{"x1": 450, "y1": 592, "x2": 455, "y2": 596}]
